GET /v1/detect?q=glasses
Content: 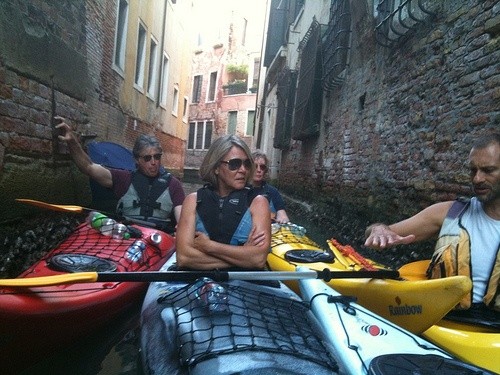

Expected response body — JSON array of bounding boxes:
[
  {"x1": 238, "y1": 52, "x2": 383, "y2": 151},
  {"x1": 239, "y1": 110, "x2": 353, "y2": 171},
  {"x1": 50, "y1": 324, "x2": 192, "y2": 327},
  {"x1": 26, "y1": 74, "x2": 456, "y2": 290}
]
[
  {"x1": 254, "y1": 163, "x2": 266, "y2": 170},
  {"x1": 220, "y1": 158, "x2": 254, "y2": 170},
  {"x1": 138, "y1": 154, "x2": 161, "y2": 162}
]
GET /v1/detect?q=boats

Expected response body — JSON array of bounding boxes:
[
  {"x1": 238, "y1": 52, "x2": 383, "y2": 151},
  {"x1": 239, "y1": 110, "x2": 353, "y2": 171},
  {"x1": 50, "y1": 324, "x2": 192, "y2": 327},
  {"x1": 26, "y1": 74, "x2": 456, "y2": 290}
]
[
  {"x1": 139, "y1": 248, "x2": 351, "y2": 375},
  {"x1": 265, "y1": 223, "x2": 473, "y2": 336},
  {"x1": 394, "y1": 259, "x2": 499, "y2": 375},
  {"x1": 0, "y1": 211, "x2": 177, "y2": 352},
  {"x1": 295, "y1": 266, "x2": 500, "y2": 375}
]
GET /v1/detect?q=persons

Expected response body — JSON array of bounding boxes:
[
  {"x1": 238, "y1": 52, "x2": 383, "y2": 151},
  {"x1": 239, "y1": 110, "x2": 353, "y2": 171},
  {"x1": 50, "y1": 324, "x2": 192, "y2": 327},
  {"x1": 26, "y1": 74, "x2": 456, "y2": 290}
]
[
  {"x1": 53, "y1": 114, "x2": 186, "y2": 228},
  {"x1": 250, "y1": 150, "x2": 289, "y2": 223},
  {"x1": 365, "y1": 135, "x2": 500, "y2": 307},
  {"x1": 176, "y1": 135, "x2": 272, "y2": 269}
]
